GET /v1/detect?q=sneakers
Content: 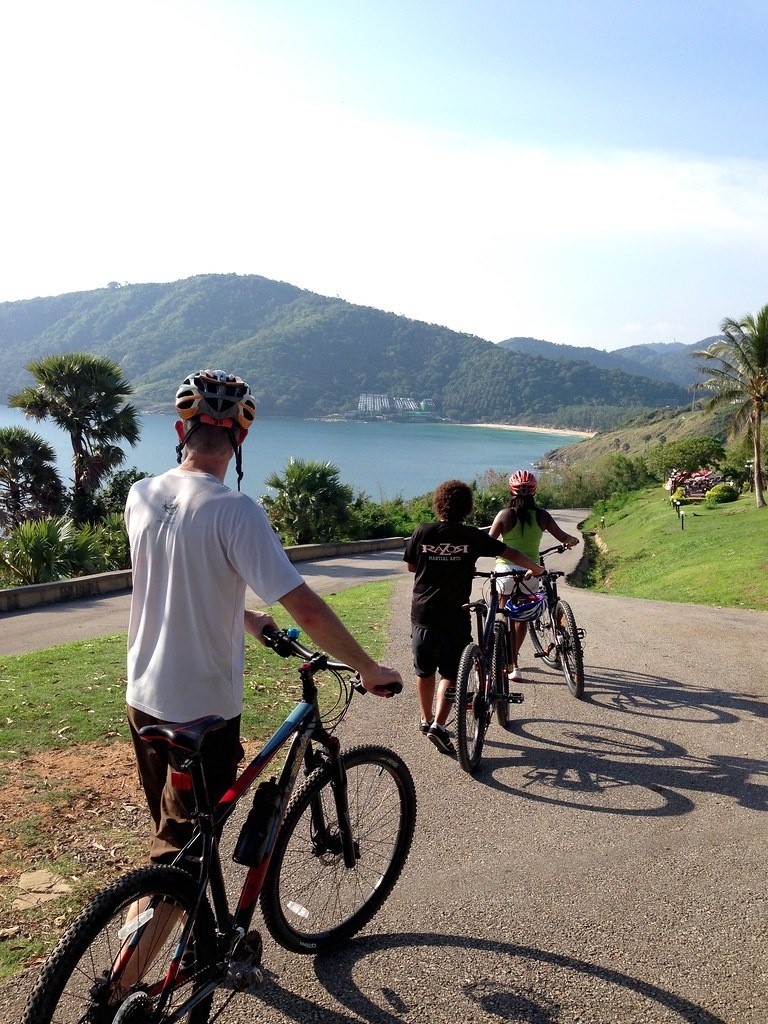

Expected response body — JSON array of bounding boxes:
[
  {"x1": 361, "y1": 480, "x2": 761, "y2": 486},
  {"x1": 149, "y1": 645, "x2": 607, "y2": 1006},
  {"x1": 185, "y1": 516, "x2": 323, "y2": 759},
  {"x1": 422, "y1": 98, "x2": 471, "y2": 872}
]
[
  {"x1": 427, "y1": 723, "x2": 456, "y2": 753},
  {"x1": 418, "y1": 716, "x2": 434, "y2": 735}
]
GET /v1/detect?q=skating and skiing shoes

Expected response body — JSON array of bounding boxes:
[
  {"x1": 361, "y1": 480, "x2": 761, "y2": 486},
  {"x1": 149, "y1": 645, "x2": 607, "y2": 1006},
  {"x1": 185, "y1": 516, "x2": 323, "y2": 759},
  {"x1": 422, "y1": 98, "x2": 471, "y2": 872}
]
[{"x1": 508, "y1": 668, "x2": 522, "y2": 680}]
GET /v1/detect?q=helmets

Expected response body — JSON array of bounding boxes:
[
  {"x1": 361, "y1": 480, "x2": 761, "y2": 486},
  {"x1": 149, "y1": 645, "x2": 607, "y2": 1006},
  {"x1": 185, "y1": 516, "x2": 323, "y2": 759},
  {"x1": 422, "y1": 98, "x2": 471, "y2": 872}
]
[
  {"x1": 504, "y1": 592, "x2": 545, "y2": 621},
  {"x1": 509, "y1": 470, "x2": 537, "y2": 496},
  {"x1": 175, "y1": 370, "x2": 255, "y2": 429}
]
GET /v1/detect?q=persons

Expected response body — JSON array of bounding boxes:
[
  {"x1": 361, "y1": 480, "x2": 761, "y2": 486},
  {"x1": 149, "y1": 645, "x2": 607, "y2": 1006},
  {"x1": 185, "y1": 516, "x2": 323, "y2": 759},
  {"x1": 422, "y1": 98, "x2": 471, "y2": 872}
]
[
  {"x1": 89, "y1": 370, "x2": 404, "y2": 1023},
  {"x1": 487, "y1": 471, "x2": 579, "y2": 681},
  {"x1": 403, "y1": 480, "x2": 545, "y2": 754}
]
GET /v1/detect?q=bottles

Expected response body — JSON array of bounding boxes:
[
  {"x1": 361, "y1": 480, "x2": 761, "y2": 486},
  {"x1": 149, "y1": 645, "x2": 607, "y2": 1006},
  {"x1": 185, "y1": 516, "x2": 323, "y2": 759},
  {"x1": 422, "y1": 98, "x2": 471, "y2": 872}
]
[{"x1": 232, "y1": 776, "x2": 279, "y2": 867}]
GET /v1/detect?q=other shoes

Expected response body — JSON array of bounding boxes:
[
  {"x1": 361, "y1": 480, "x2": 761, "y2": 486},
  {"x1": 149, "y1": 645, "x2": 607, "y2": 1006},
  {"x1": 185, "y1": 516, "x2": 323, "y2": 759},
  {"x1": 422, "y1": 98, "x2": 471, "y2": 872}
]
[
  {"x1": 89, "y1": 985, "x2": 128, "y2": 1023},
  {"x1": 181, "y1": 919, "x2": 221, "y2": 970}
]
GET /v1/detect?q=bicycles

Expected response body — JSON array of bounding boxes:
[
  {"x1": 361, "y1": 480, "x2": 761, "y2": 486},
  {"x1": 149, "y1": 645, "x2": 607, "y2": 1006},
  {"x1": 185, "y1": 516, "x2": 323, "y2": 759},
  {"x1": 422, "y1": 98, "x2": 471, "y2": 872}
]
[
  {"x1": 444, "y1": 569, "x2": 548, "y2": 773},
  {"x1": 20, "y1": 625, "x2": 416, "y2": 1024},
  {"x1": 526, "y1": 543, "x2": 587, "y2": 698}
]
